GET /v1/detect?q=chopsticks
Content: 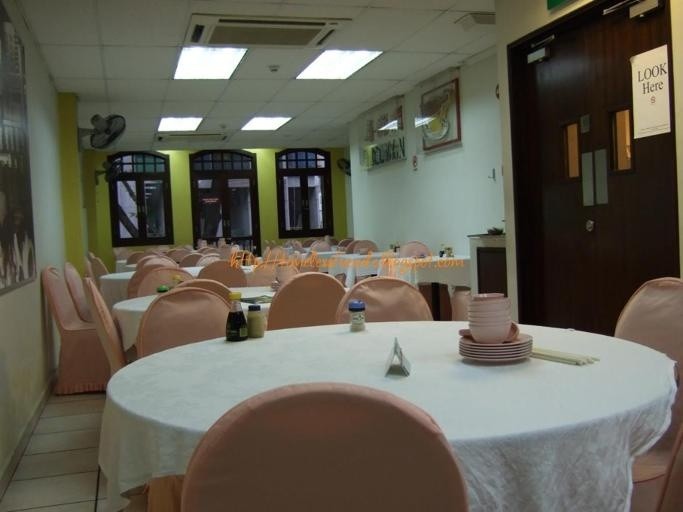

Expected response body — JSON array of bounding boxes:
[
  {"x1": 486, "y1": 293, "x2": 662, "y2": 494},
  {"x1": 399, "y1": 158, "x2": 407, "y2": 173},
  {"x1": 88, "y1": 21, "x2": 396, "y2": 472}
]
[{"x1": 532, "y1": 346, "x2": 600, "y2": 366}]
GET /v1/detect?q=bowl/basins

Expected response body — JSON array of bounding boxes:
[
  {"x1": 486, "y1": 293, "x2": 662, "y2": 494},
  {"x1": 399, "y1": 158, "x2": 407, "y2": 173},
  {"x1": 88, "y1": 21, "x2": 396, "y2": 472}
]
[{"x1": 466, "y1": 292, "x2": 512, "y2": 344}]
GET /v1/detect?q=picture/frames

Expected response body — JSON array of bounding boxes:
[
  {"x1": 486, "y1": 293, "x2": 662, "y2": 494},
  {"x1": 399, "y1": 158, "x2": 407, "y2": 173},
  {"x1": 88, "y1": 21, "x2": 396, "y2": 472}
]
[
  {"x1": 421, "y1": 78, "x2": 462, "y2": 154},
  {"x1": 356, "y1": 104, "x2": 405, "y2": 169},
  {"x1": 0, "y1": 1, "x2": 37, "y2": 295}
]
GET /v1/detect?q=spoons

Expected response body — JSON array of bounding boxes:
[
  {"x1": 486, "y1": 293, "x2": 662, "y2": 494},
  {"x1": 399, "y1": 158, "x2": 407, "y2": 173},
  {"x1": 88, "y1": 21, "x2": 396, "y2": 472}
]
[{"x1": 458, "y1": 321, "x2": 519, "y2": 343}]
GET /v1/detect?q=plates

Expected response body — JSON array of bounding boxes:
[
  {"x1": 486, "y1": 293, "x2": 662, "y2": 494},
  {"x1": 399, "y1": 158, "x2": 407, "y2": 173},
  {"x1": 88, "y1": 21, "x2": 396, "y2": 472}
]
[
  {"x1": 422, "y1": 115, "x2": 449, "y2": 141},
  {"x1": 458, "y1": 334, "x2": 533, "y2": 363}
]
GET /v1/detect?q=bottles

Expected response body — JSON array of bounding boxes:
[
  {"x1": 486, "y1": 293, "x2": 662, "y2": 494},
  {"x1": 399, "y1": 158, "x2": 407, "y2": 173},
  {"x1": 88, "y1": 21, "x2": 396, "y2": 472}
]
[
  {"x1": 393, "y1": 240, "x2": 401, "y2": 254},
  {"x1": 439, "y1": 243, "x2": 450, "y2": 257},
  {"x1": 246, "y1": 304, "x2": 265, "y2": 338},
  {"x1": 226, "y1": 291, "x2": 250, "y2": 342},
  {"x1": 348, "y1": 300, "x2": 366, "y2": 332},
  {"x1": 156, "y1": 275, "x2": 180, "y2": 296}
]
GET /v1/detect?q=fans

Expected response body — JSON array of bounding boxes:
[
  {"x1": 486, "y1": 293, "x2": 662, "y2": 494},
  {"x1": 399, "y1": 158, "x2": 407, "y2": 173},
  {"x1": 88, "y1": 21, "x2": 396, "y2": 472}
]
[
  {"x1": 95, "y1": 160, "x2": 121, "y2": 185},
  {"x1": 77, "y1": 113, "x2": 125, "y2": 150},
  {"x1": 337, "y1": 157, "x2": 351, "y2": 177}
]
